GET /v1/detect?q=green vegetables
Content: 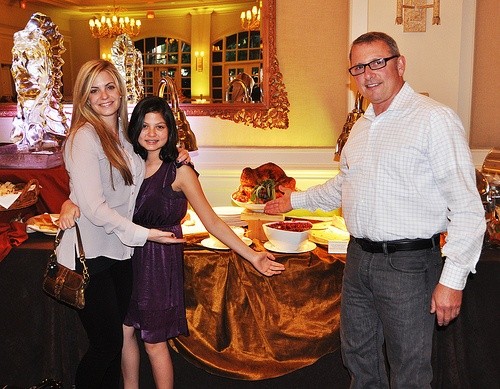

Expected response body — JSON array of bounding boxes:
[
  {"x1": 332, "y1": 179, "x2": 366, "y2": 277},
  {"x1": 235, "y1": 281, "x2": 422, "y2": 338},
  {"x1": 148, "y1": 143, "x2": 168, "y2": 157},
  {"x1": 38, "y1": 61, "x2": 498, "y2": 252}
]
[{"x1": 248, "y1": 179, "x2": 276, "y2": 202}]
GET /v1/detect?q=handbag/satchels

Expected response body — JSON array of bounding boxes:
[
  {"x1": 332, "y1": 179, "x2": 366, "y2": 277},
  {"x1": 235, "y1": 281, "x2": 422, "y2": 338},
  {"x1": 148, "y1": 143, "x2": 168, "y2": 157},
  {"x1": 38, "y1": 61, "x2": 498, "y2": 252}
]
[{"x1": 41, "y1": 220, "x2": 90, "y2": 310}]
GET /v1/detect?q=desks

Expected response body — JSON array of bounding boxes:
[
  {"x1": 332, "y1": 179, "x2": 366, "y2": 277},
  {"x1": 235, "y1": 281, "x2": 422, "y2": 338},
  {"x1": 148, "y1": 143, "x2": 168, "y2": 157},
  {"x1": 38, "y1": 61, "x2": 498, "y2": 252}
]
[{"x1": 0, "y1": 143, "x2": 69, "y2": 229}]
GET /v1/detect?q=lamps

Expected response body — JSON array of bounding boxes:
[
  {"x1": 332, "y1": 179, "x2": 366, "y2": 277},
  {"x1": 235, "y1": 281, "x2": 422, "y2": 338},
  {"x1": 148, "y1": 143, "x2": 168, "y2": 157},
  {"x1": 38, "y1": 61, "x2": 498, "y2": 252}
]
[
  {"x1": 240, "y1": 5, "x2": 261, "y2": 31},
  {"x1": 195, "y1": 51, "x2": 205, "y2": 73},
  {"x1": 88, "y1": 0, "x2": 142, "y2": 39}
]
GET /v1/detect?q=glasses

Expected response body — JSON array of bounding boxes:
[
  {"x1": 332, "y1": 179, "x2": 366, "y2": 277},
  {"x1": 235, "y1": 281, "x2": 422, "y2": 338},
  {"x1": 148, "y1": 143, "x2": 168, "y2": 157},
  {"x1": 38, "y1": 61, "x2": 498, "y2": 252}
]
[{"x1": 348, "y1": 56, "x2": 399, "y2": 76}]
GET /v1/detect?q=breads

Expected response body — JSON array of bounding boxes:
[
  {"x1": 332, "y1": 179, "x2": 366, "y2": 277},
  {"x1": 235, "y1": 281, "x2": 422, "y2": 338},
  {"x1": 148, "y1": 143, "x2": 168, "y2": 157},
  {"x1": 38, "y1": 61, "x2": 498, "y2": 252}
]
[
  {"x1": 0, "y1": 182, "x2": 16, "y2": 196},
  {"x1": 34, "y1": 214, "x2": 59, "y2": 231},
  {"x1": 181, "y1": 212, "x2": 195, "y2": 226}
]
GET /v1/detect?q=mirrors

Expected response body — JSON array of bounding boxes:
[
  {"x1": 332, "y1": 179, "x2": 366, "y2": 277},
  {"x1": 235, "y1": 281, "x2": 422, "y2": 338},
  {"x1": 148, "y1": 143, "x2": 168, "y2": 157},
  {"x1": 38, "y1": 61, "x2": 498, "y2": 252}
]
[{"x1": 0, "y1": 0, "x2": 290, "y2": 130}]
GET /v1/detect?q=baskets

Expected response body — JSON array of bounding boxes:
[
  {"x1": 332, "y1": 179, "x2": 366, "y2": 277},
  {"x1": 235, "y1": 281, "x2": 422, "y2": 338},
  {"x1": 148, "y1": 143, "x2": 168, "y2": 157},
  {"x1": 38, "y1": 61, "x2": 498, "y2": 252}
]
[{"x1": 0, "y1": 178, "x2": 40, "y2": 211}]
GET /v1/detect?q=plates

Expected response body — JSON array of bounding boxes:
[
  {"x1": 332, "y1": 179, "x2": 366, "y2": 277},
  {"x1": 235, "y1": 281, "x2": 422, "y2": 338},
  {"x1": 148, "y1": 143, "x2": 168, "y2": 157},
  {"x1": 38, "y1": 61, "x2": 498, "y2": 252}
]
[
  {"x1": 28, "y1": 213, "x2": 61, "y2": 236},
  {"x1": 200, "y1": 236, "x2": 253, "y2": 250},
  {"x1": 264, "y1": 240, "x2": 317, "y2": 254},
  {"x1": 308, "y1": 237, "x2": 349, "y2": 249}
]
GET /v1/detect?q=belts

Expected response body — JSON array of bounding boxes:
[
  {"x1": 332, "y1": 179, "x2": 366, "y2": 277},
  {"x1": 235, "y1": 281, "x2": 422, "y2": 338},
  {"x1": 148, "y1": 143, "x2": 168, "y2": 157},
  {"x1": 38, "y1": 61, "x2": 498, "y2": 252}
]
[{"x1": 357, "y1": 237, "x2": 439, "y2": 253}]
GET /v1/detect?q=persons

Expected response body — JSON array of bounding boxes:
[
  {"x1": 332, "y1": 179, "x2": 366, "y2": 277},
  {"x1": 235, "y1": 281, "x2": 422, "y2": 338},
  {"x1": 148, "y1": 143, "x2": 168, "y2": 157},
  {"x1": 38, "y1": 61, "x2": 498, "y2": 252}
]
[
  {"x1": 264, "y1": 32, "x2": 487, "y2": 389},
  {"x1": 57, "y1": 96, "x2": 286, "y2": 389},
  {"x1": 54, "y1": 58, "x2": 192, "y2": 389}
]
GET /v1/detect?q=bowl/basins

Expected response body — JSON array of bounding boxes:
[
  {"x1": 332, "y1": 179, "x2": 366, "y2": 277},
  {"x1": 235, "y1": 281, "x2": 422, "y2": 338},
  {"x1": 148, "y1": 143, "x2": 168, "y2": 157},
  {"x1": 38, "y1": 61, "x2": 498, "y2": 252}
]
[
  {"x1": 230, "y1": 191, "x2": 266, "y2": 213},
  {"x1": 208, "y1": 226, "x2": 244, "y2": 247},
  {"x1": 213, "y1": 206, "x2": 245, "y2": 222},
  {"x1": 262, "y1": 220, "x2": 313, "y2": 251}
]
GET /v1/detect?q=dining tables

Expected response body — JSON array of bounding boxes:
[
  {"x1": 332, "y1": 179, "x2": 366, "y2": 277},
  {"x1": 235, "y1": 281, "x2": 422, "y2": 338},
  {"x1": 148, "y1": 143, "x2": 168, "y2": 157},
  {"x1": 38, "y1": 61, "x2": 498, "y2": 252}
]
[{"x1": 13, "y1": 209, "x2": 500, "y2": 389}]
